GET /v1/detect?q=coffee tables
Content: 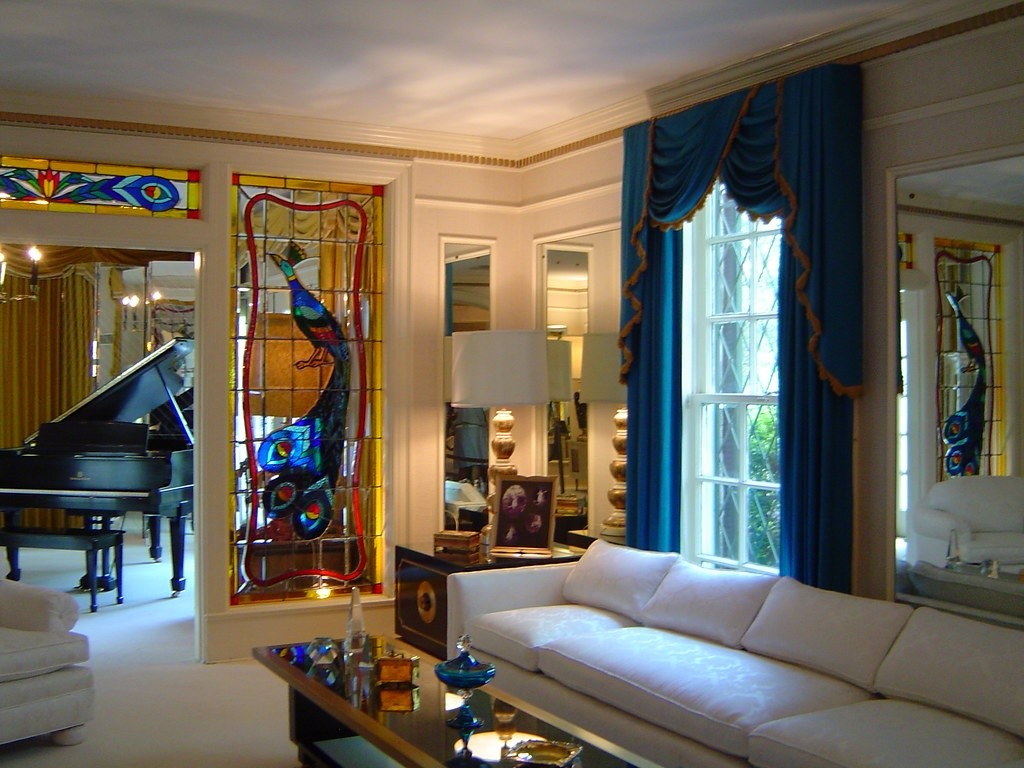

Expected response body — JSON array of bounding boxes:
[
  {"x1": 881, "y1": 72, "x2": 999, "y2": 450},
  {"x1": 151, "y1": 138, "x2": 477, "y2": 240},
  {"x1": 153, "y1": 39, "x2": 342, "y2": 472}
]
[{"x1": 249, "y1": 636, "x2": 667, "y2": 768}]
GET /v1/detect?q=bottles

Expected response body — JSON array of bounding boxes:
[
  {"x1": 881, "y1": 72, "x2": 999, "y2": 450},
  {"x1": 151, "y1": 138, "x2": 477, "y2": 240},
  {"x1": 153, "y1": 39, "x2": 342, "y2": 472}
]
[
  {"x1": 946, "y1": 529, "x2": 960, "y2": 568},
  {"x1": 344, "y1": 587, "x2": 367, "y2": 652}
]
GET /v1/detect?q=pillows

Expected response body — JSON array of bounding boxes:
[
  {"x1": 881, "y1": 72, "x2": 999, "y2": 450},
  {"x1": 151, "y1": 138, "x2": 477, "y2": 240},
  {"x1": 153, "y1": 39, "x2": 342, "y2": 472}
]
[
  {"x1": 876, "y1": 605, "x2": 1024, "y2": 739},
  {"x1": 639, "y1": 554, "x2": 781, "y2": 645},
  {"x1": 740, "y1": 575, "x2": 915, "y2": 693},
  {"x1": 562, "y1": 538, "x2": 677, "y2": 621}
]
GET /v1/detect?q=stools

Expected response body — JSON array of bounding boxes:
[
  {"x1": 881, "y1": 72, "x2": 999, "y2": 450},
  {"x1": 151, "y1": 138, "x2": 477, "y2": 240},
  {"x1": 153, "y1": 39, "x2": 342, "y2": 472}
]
[{"x1": 0, "y1": 525, "x2": 127, "y2": 612}]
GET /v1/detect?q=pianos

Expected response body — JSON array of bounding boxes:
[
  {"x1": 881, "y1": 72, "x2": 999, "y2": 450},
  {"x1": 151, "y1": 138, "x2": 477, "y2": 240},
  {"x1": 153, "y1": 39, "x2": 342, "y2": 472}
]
[{"x1": 0, "y1": 336, "x2": 193, "y2": 610}]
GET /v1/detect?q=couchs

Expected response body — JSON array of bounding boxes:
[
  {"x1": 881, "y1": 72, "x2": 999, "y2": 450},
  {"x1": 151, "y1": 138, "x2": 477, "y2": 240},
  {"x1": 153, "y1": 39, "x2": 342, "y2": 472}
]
[
  {"x1": 0, "y1": 576, "x2": 97, "y2": 748},
  {"x1": 904, "y1": 474, "x2": 1024, "y2": 567},
  {"x1": 446, "y1": 559, "x2": 1024, "y2": 768}
]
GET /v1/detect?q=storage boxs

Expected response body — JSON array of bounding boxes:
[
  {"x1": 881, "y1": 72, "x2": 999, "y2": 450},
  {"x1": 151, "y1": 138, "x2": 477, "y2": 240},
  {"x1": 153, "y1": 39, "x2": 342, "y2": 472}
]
[
  {"x1": 374, "y1": 683, "x2": 420, "y2": 714},
  {"x1": 432, "y1": 529, "x2": 480, "y2": 553},
  {"x1": 374, "y1": 654, "x2": 421, "y2": 682}
]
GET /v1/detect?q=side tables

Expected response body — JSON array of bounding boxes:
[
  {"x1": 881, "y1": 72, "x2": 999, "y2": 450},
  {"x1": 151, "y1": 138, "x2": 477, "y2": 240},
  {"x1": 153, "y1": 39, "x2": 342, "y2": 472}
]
[
  {"x1": 395, "y1": 541, "x2": 583, "y2": 664},
  {"x1": 568, "y1": 529, "x2": 628, "y2": 549}
]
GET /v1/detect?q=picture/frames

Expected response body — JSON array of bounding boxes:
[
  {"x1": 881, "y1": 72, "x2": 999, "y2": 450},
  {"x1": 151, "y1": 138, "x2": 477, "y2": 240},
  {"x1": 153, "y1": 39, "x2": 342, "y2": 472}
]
[{"x1": 491, "y1": 473, "x2": 559, "y2": 553}]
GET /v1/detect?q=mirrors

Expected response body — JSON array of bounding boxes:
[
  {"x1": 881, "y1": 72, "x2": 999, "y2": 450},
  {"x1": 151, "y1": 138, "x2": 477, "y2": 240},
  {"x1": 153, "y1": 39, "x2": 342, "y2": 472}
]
[
  {"x1": 527, "y1": 221, "x2": 628, "y2": 547},
  {"x1": 438, "y1": 233, "x2": 497, "y2": 544},
  {"x1": 883, "y1": 142, "x2": 1024, "y2": 631}
]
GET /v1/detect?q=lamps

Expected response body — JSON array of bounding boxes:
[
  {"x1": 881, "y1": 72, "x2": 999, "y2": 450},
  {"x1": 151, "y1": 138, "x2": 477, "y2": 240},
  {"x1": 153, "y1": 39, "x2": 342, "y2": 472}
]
[
  {"x1": 547, "y1": 338, "x2": 573, "y2": 402},
  {"x1": 453, "y1": 326, "x2": 552, "y2": 545},
  {"x1": 0, "y1": 245, "x2": 42, "y2": 304},
  {"x1": 578, "y1": 331, "x2": 628, "y2": 537}
]
